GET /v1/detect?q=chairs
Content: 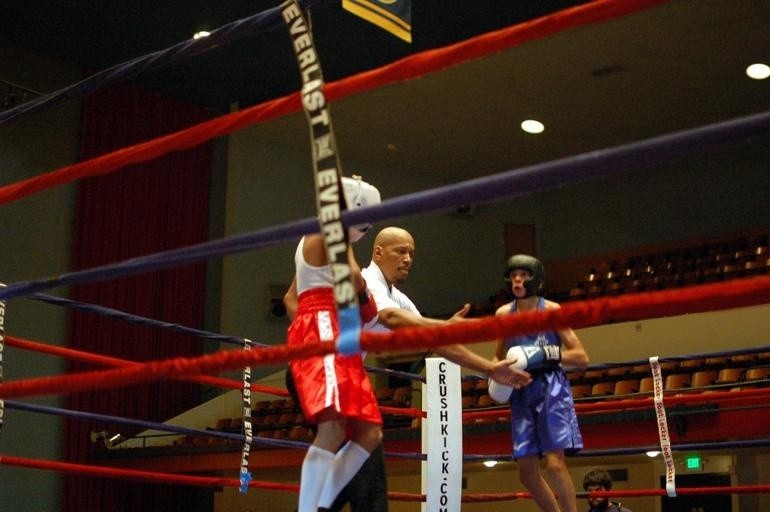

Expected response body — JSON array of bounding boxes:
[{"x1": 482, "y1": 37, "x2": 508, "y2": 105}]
[
  {"x1": 568, "y1": 233, "x2": 770, "y2": 301},
  {"x1": 176, "y1": 347, "x2": 770, "y2": 450}
]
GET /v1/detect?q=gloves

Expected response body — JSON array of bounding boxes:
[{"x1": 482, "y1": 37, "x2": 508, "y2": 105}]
[
  {"x1": 506, "y1": 344, "x2": 561, "y2": 372},
  {"x1": 358, "y1": 278, "x2": 379, "y2": 331},
  {"x1": 488, "y1": 375, "x2": 514, "y2": 404}
]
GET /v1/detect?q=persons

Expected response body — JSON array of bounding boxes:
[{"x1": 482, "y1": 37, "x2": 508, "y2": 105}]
[
  {"x1": 349, "y1": 225, "x2": 531, "y2": 512},
  {"x1": 579, "y1": 470, "x2": 629, "y2": 512},
  {"x1": 482, "y1": 253, "x2": 590, "y2": 510},
  {"x1": 284, "y1": 172, "x2": 385, "y2": 512}
]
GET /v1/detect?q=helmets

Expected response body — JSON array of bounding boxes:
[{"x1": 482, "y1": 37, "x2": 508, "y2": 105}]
[
  {"x1": 584, "y1": 471, "x2": 610, "y2": 490},
  {"x1": 339, "y1": 178, "x2": 381, "y2": 245},
  {"x1": 505, "y1": 254, "x2": 544, "y2": 299}
]
